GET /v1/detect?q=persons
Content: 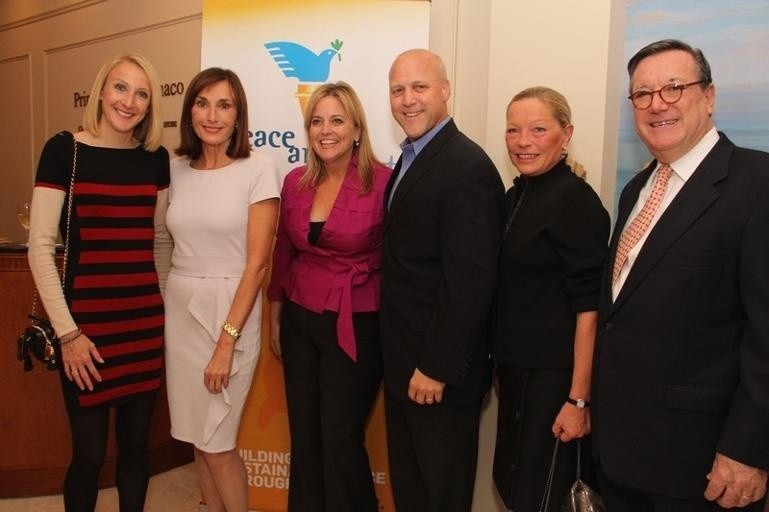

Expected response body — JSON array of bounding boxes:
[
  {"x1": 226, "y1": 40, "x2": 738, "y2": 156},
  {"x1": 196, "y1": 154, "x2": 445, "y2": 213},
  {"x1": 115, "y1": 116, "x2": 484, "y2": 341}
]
[
  {"x1": 384, "y1": 49, "x2": 508, "y2": 511},
  {"x1": 606, "y1": 39, "x2": 768, "y2": 511},
  {"x1": 488, "y1": 85, "x2": 610, "y2": 511},
  {"x1": 169, "y1": 67, "x2": 281, "y2": 511},
  {"x1": 266, "y1": 82, "x2": 393, "y2": 511},
  {"x1": 16, "y1": 49, "x2": 175, "y2": 511}
]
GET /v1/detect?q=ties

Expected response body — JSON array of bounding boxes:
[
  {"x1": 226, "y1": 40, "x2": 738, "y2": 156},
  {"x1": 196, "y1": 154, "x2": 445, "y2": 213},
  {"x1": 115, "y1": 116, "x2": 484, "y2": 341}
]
[{"x1": 610, "y1": 165, "x2": 674, "y2": 287}]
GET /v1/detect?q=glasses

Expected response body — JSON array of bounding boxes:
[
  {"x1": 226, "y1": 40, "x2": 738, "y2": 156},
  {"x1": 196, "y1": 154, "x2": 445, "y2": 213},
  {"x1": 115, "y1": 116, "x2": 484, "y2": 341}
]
[{"x1": 627, "y1": 79, "x2": 708, "y2": 110}]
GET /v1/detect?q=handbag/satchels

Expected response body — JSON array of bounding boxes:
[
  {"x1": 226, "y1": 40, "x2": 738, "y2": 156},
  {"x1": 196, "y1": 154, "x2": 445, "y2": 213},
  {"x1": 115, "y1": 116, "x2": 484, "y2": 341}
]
[
  {"x1": 561, "y1": 479, "x2": 608, "y2": 512},
  {"x1": 17, "y1": 319, "x2": 62, "y2": 371}
]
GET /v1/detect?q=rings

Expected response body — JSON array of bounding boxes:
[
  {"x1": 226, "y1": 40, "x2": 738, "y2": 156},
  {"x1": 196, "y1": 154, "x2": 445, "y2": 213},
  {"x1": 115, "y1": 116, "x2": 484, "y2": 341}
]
[
  {"x1": 71, "y1": 369, "x2": 77, "y2": 374},
  {"x1": 741, "y1": 495, "x2": 753, "y2": 498},
  {"x1": 427, "y1": 398, "x2": 434, "y2": 402}
]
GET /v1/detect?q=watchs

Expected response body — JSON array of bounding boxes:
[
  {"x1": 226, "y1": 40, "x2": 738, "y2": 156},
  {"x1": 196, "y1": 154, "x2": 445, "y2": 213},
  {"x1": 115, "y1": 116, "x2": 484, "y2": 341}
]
[{"x1": 567, "y1": 397, "x2": 591, "y2": 408}]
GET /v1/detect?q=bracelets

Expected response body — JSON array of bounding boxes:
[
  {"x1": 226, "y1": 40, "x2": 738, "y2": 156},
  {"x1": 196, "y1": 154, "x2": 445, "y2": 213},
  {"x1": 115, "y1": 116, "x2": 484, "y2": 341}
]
[
  {"x1": 221, "y1": 319, "x2": 241, "y2": 341},
  {"x1": 57, "y1": 327, "x2": 82, "y2": 344}
]
